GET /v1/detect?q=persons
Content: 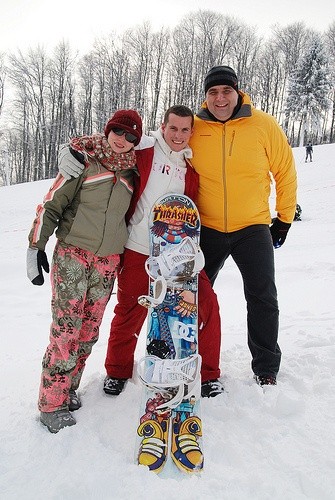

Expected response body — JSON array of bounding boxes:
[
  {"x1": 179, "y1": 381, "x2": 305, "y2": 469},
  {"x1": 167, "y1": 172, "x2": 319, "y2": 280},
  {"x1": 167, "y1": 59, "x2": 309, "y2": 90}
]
[
  {"x1": 305, "y1": 141, "x2": 313, "y2": 163},
  {"x1": 56, "y1": 105, "x2": 224, "y2": 398},
  {"x1": 187, "y1": 66, "x2": 297, "y2": 398},
  {"x1": 26, "y1": 109, "x2": 143, "y2": 433}
]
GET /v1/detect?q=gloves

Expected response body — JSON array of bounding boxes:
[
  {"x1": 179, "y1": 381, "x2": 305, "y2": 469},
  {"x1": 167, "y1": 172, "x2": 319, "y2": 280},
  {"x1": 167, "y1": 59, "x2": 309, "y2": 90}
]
[
  {"x1": 58, "y1": 147, "x2": 86, "y2": 179},
  {"x1": 269, "y1": 218, "x2": 291, "y2": 248},
  {"x1": 26, "y1": 247, "x2": 50, "y2": 286}
]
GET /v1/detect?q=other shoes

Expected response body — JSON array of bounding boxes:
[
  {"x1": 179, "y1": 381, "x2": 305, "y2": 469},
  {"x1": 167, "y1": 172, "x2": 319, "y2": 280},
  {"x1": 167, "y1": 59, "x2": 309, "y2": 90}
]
[
  {"x1": 200, "y1": 379, "x2": 225, "y2": 399},
  {"x1": 255, "y1": 376, "x2": 277, "y2": 389},
  {"x1": 103, "y1": 375, "x2": 127, "y2": 396},
  {"x1": 38, "y1": 409, "x2": 78, "y2": 434},
  {"x1": 65, "y1": 390, "x2": 82, "y2": 411}
]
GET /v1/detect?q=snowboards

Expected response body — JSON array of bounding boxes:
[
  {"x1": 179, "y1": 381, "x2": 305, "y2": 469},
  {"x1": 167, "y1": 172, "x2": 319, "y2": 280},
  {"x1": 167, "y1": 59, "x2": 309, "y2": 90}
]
[{"x1": 136, "y1": 192, "x2": 202, "y2": 455}]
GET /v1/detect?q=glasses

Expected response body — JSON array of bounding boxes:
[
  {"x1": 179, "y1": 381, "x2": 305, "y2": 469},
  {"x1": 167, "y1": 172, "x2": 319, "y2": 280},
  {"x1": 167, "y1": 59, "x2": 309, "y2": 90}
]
[{"x1": 112, "y1": 127, "x2": 139, "y2": 146}]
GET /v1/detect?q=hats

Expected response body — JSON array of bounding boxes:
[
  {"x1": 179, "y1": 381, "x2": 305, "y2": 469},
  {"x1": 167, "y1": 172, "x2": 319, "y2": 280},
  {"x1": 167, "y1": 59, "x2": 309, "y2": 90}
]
[
  {"x1": 205, "y1": 65, "x2": 238, "y2": 96},
  {"x1": 104, "y1": 109, "x2": 143, "y2": 147}
]
